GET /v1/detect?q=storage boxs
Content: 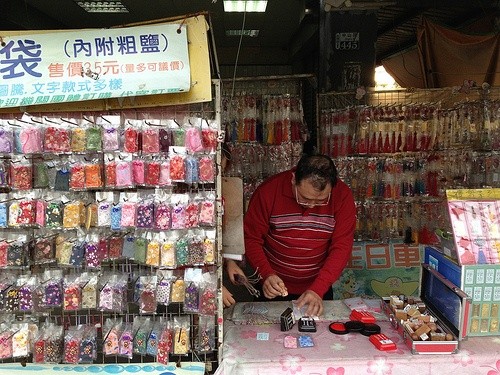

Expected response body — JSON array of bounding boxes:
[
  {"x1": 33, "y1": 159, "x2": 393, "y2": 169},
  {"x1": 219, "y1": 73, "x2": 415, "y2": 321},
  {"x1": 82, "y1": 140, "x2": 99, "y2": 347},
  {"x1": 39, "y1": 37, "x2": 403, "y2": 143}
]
[{"x1": 422, "y1": 246, "x2": 500, "y2": 336}]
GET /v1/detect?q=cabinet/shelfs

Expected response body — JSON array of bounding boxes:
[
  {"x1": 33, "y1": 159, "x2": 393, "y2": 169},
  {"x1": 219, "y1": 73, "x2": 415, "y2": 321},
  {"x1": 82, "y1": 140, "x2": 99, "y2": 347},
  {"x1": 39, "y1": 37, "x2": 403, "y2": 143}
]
[{"x1": 0, "y1": 76, "x2": 500, "y2": 375}]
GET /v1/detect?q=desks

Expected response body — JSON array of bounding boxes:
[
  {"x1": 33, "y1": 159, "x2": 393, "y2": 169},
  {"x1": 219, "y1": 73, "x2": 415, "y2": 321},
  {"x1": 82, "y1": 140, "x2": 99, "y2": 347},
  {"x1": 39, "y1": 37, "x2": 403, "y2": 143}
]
[{"x1": 221, "y1": 298, "x2": 500, "y2": 375}]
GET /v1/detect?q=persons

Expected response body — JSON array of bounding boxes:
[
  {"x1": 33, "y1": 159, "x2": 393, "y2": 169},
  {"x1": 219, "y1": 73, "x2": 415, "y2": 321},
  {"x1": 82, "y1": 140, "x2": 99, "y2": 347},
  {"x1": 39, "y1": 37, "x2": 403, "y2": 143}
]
[
  {"x1": 243, "y1": 153, "x2": 355, "y2": 317},
  {"x1": 221, "y1": 149, "x2": 247, "y2": 310}
]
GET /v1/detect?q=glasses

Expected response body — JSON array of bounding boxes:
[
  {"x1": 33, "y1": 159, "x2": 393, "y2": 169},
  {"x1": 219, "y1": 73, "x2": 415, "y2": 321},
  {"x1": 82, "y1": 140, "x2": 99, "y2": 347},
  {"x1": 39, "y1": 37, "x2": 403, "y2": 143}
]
[{"x1": 295, "y1": 185, "x2": 331, "y2": 207}]
